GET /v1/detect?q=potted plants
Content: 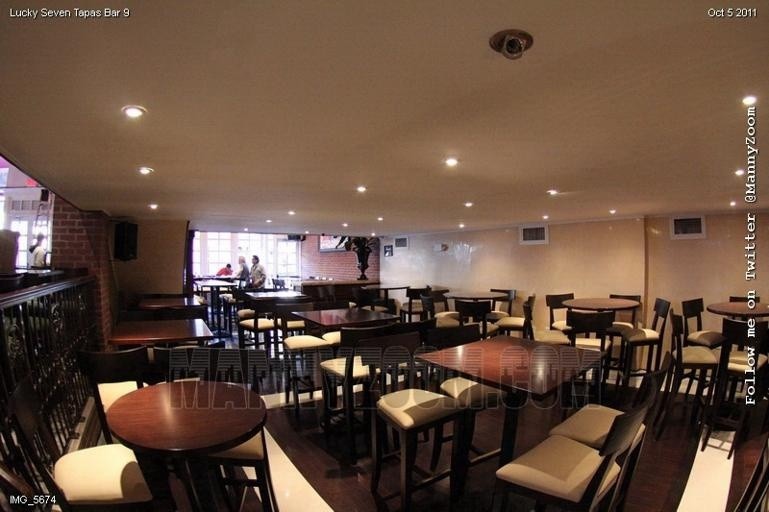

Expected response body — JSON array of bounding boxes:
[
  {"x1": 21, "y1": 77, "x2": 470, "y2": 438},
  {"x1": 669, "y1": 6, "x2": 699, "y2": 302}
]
[{"x1": 333, "y1": 236, "x2": 382, "y2": 280}]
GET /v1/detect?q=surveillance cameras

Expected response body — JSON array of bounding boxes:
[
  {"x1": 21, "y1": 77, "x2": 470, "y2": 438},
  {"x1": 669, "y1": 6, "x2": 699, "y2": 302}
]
[{"x1": 500, "y1": 35, "x2": 528, "y2": 62}]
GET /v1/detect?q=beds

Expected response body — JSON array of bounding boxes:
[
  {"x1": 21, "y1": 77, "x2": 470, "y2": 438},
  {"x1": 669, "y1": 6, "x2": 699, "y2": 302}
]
[{"x1": 706, "y1": 302, "x2": 768, "y2": 351}]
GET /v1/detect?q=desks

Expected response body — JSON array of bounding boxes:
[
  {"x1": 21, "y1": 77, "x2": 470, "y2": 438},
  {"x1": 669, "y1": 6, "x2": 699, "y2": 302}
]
[{"x1": 301, "y1": 279, "x2": 380, "y2": 310}]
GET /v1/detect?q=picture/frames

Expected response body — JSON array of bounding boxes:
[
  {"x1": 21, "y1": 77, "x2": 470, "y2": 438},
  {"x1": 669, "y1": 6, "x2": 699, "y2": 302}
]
[{"x1": 384, "y1": 245, "x2": 393, "y2": 257}]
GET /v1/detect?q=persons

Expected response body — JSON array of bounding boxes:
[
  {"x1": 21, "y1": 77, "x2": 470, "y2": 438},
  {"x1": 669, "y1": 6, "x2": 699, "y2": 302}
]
[
  {"x1": 216, "y1": 264, "x2": 232, "y2": 275},
  {"x1": 251, "y1": 255, "x2": 267, "y2": 288},
  {"x1": 28, "y1": 234, "x2": 45, "y2": 266},
  {"x1": 237, "y1": 256, "x2": 249, "y2": 284}
]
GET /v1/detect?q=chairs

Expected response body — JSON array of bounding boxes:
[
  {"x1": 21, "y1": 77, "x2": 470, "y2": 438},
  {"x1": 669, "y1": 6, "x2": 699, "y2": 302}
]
[
  {"x1": 546, "y1": 293, "x2": 575, "y2": 335},
  {"x1": 84, "y1": 346, "x2": 149, "y2": 445},
  {"x1": 239, "y1": 298, "x2": 280, "y2": 350},
  {"x1": 548, "y1": 351, "x2": 672, "y2": 512},
  {"x1": 711, "y1": 318, "x2": 769, "y2": 408},
  {"x1": 387, "y1": 317, "x2": 437, "y2": 376},
  {"x1": 276, "y1": 302, "x2": 330, "y2": 404},
  {"x1": 490, "y1": 289, "x2": 517, "y2": 320},
  {"x1": 203, "y1": 350, "x2": 279, "y2": 512},
  {"x1": 400, "y1": 288, "x2": 432, "y2": 322},
  {"x1": 682, "y1": 297, "x2": 728, "y2": 349},
  {"x1": 522, "y1": 301, "x2": 571, "y2": 346},
  {"x1": 358, "y1": 332, "x2": 464, "y2": 512},
  {"x1": 153, "y1": 341, "x2": 226, "y2": 381},
  {"x1": 426, "y1": 285, "x2": 460, "y2": 318},
  {"x1": 496, "y1": 293, "x2": 536, "y2": 335},
  {"x1": 320, "y1": 323, "x2": 401, "y2": 428},
  {"x1": 491, "y1": 377, "x2": 657, "y2": 512},
  {"x1": 454, "y1": 300, "x2": 492, "y2": 340},
  {"x1": 662, "y1": 308, "x2": 718, "y2": 405},
  {"x1": 566, "y1": 311, "x2": 613, "y2": 357},
  {"x1": 601, "y1": 294, "x2": 641, "y2": 367},
  {"x1": 420, "y1": 293, "x2": 459, "y2": 328},
  {"x1": 424, "y1": 324, "x2": 507, "y2": 488},
  {"x1": 618, "y1": 298, "x2": 671, "y2": 388},
  {"x1": 8, "y1": 374, "x2": 153, "y2": 511}
]
